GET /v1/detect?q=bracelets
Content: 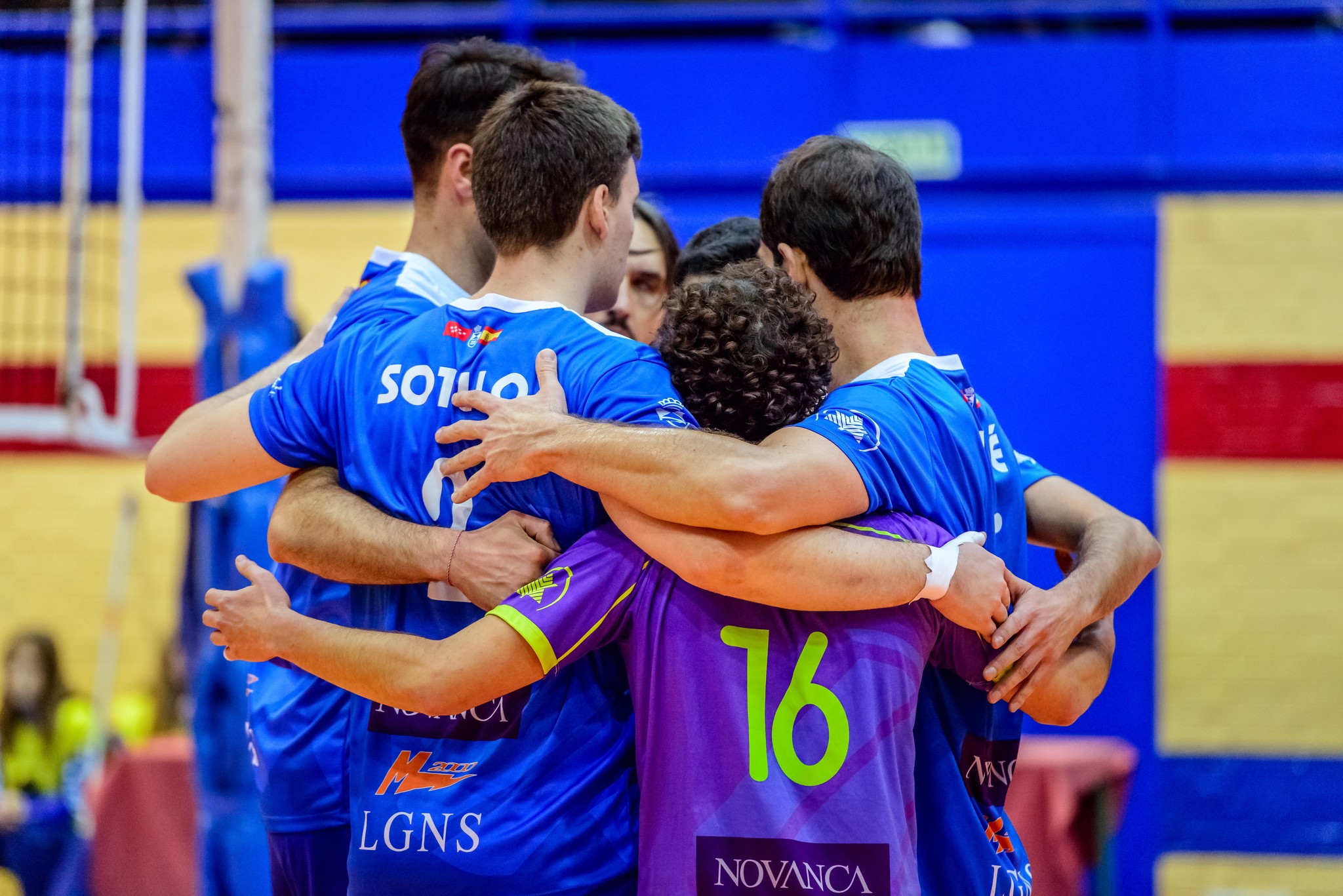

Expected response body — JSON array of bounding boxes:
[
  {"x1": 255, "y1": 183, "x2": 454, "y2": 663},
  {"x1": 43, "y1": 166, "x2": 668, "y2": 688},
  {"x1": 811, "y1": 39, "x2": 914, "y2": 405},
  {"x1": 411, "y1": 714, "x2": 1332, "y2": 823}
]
[{"x1": 445, "y1": 529, "x2": 465, "y2": 585}]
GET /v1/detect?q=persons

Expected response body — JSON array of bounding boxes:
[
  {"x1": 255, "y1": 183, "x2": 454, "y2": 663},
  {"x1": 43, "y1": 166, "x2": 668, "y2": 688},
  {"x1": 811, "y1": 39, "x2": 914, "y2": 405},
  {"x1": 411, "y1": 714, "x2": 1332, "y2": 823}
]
[
  {"x1": 144, "y1": 30, "x2": 1166, "y2": 896},
  {"x1": 0, "y1": 630, "x2": 113, "y2": 896}
]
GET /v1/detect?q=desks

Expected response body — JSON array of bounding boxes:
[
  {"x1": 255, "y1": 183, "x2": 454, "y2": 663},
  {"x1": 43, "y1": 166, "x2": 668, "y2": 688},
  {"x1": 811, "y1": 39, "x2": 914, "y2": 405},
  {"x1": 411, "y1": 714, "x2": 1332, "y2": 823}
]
[{"x1": 90, "y1": 733, "x2": 1139, "y2": 896}]
